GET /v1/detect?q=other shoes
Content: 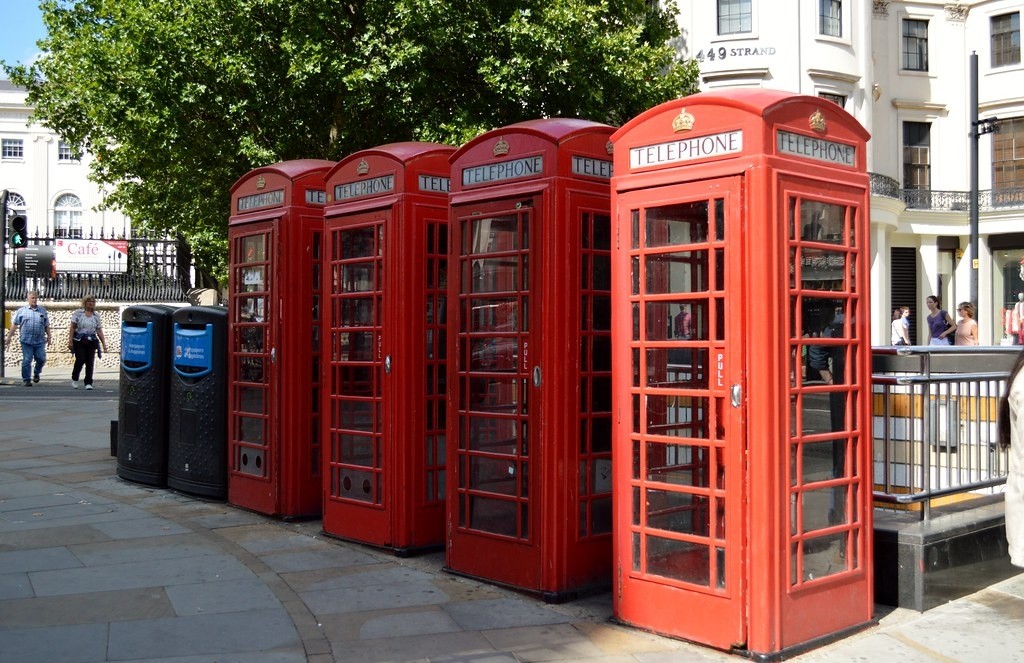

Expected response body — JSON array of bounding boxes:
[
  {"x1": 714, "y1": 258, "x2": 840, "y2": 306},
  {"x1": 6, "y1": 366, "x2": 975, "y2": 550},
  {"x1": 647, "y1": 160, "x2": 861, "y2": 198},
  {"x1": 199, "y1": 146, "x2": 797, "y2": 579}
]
[
  {"x1": 33, "y1": 370, "x2": 40, "y2": 383},
  {"x1": 83, "y1": 382, "x2": 93, "y2": 389},
  {"x1": 71, "y1": 377, "x2": 79, "y2": 388},
  {"x1": 23, "y1": 379, "x2": 32, "y2": 386}
]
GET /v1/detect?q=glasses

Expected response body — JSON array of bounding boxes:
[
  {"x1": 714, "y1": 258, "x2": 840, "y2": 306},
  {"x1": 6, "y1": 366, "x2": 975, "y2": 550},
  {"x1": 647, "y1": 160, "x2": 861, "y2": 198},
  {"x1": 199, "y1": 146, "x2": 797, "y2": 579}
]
[{"x1": 86, "y1": 300, "x2": 94, "y2": 303}]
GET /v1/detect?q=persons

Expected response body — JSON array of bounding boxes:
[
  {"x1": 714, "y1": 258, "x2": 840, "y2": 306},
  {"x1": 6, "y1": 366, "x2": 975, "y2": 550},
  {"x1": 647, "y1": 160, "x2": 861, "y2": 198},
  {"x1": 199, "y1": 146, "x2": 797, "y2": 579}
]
[
  {"x1": 4, "y1": 291, "x2": 51, "y2": 387},
  {"x1": 809, "y1": 312, "x2": 855, "y2": 525},
  {"x1": 926, "y1": 295, "x2": 956, "y2": 346},
  {"x1": 67, "y1": 294, "x2": 108, "y2": 390},
  {"x1": 1015, "y1": 292, "x2": 1024, "y2": 345},
  {"x1": 955, "y1": 301, "x2": 978, "y2": 346},
  {"x1": 674, "y1": 303, "x2": 691, "y2": 340},
  {"x1": 997, "y1": 350, "x2": 1024, "y2": 567},
  {"x1": 891, "y1": 306, "x2": 911, "y2": 347}
]
